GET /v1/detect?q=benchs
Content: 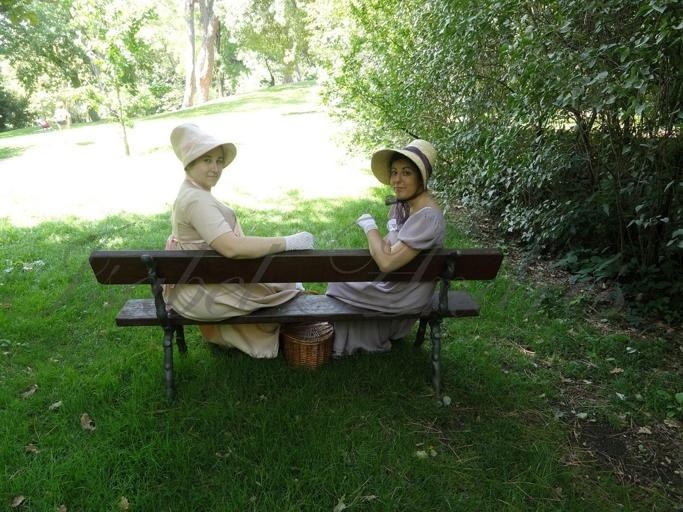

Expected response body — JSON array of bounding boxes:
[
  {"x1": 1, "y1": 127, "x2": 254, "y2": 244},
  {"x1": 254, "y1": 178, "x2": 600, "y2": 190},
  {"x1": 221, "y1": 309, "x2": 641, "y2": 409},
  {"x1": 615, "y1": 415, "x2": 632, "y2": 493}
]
[{"x1": 87, "y1": 250, "x2": 503, "y2": 407}]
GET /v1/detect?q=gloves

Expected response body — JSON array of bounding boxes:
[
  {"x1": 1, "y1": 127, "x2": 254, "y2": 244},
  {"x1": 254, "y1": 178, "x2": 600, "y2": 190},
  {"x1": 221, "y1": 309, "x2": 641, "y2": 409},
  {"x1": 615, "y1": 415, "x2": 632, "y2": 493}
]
[
  {"x1": 284, "y1": 230, "x2": 316, "y2": 252},
  {"x1": 355, "y1": 212, "x2": 378, "y2": 234}
]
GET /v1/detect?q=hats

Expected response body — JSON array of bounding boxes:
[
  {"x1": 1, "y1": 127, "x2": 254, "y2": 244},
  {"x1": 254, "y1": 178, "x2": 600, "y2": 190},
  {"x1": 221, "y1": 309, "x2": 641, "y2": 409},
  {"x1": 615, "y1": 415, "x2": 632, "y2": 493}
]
[
  {"x1": 370, "y1": 139, "x2": 438, "y2": 191},
  {"x1": 170, "y1": 122, "x2": 237, "y2": 171}
]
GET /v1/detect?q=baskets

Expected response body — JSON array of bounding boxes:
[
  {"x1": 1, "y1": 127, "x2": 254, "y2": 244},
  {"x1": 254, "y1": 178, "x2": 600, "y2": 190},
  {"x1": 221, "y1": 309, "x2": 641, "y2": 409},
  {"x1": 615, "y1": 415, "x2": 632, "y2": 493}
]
[{"x1": 280, "y1": 320, "x2": 336, "y2": 368}]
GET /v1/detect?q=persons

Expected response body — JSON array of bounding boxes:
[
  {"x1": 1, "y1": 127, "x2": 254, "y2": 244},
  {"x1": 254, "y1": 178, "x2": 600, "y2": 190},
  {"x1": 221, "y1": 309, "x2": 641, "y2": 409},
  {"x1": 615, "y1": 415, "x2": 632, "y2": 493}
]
[
  {"x1": 325, "y1": 140, "x2": 445, "y2": 358},
  {"x1": 161, "y1": 121, "x2": 314, "y2": 359},
  {"x1": 54, "y1": 101, "x2": 71, "y2": 131}
]
